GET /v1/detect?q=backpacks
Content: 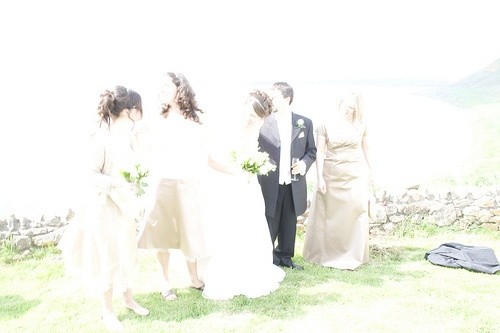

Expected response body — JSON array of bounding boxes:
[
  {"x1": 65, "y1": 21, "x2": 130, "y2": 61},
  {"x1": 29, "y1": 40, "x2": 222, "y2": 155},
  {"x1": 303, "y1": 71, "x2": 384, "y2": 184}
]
[{"x1": 425, "y1": 242, "x2": 500, "y2": 275}]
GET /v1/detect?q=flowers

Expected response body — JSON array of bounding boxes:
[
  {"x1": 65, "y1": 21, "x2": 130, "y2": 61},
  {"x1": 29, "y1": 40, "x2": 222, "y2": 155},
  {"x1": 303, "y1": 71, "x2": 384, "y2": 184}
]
[
  {"x1": 296, "y1": 118, "x2": 306, "y2": 129},
  {"x1": 124, "y1": 164, "x2": 151, "y2": 199},
  {"x1": 240, "y1": 150, "x2": 277, "y2": 177}
]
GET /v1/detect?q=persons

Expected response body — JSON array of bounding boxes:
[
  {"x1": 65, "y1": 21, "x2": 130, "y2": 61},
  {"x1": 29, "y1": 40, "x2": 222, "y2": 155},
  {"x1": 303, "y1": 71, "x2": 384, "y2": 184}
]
[
  {"x1": 258, "y1": 81, "x2": 318, "y2": 270},
  {"x1": 201, "y1": 89, "x2": 284, "y2": 300},
  {"x1": 302, "y1": 91, "x2": 376, "y2": 271},
  {"x1": 139, "y1": 71, "x2": 209, "y2": 301},
  {"x1": 65, "y1": 86, "x2": 151, "y2": 331}
]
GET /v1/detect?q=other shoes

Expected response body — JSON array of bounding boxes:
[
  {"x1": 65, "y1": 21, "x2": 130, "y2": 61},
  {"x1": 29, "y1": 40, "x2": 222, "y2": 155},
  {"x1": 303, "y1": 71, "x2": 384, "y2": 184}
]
[
  {"x1": 189, "y1": 280, "x2": 205, "y2": 291},
  {"x1": 103, "y1": 306, "x2": 122, "y2": 331},
  {"x1": 160, "y1": 286, "x2": 177, "y2": 301},
  {"x1": 124, "y1": 299, "x2": 149, "y2": 315},
  {"x1": 290, "y1": 263, "x2": 305, "y2": 272}
]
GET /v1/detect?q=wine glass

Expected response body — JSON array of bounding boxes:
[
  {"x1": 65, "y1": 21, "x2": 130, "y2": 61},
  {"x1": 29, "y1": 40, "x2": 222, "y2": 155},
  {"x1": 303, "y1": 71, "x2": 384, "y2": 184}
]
[{"x1": 292, "y1": 156, "x2": 300, "y2": 181}]
